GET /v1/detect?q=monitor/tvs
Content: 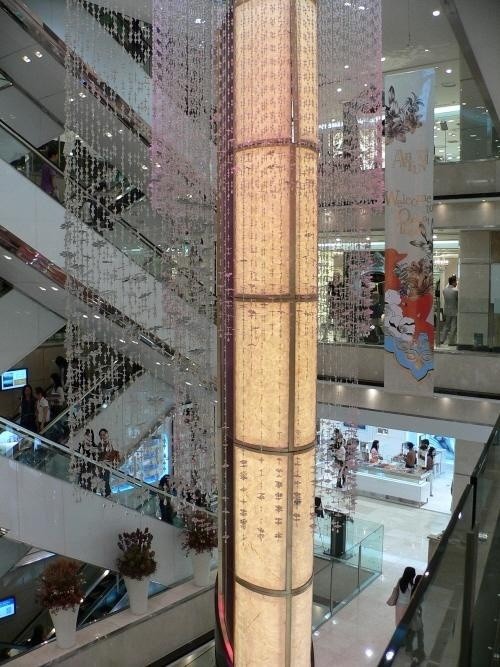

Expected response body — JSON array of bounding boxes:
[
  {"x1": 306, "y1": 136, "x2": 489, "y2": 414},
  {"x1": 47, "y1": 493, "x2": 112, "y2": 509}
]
[
  {"x1": 0, "y1": 594, "x2": 17, "y2": 620},
  {"x1": 1, "y1": 368, "x2": 29, "y2": 391}
]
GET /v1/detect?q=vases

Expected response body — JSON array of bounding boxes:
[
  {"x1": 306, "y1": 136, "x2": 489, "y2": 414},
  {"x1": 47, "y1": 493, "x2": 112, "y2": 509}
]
[
  {"x1": 123, "y1": 574, "x2": 150, "y2": 615},
  {"x1": 48, "y1": 602, "x2": 80, "y2": 649},
  {"x1": 191, "y1": 549, "x2": 213, "y2": 586}
]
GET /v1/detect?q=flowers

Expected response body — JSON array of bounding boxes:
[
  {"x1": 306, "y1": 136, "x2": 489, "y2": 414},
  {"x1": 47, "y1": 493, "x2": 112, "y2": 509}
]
[
  {"x1": 116, "y1": 527, "x2": 158, "y2": 581},
  {"x1": 36, "y1": 553, "x2": 90, "y2": 615},
  {"x1": 180, "y1": 509, "x2": 219, "y2": 560}
]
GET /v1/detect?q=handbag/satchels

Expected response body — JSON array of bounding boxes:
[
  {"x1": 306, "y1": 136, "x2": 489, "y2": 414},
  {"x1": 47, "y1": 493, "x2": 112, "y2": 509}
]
[{"x1": 386, "y1": 580, "x2": 401, "y2": 605}]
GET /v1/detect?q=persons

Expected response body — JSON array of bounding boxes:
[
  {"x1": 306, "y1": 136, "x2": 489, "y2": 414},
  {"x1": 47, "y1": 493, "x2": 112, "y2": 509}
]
[
  {"x1": 98, "y1": 429, "x2": 117, "y2": 499},
  {"x1": 407, "y1": 575, "x2": 427, "y2": 655},
  {"x1": 387, "y1": 566, "x2": 414, "y2": 646},
  {"x1": 404, "y1": 436, "x2": 436, "y2": 499},
  {"x1": 319, "y1": 272, "x2": 345, "y2": 342},
  {"x1": 78, "y1": 201, "x2": 96, "y2": 228},
  {"x1": 440, "y1": 274, "x2": 459, "y2": 346},
  {"x1": 18, "y1": 348, "x2": 87, "y2": 435},
  {"x1": 25, "y1": 623, "x2": 45, "y2": 648},
  {"x1": 158, "y1": 474, "x2": 174, "y2": 526},
  {"x1": 369, "y1": 440, "x2": 383, "y2": 464},
  {"x1": 76, "y1": 428, "x2": 96, "y2": 491},
  {"x1": 39, "y1": 146, "x2": 59, "y2": 204},
  {"x1": 329, "y1": 428, "x2": 361, "y2": 487}
]
